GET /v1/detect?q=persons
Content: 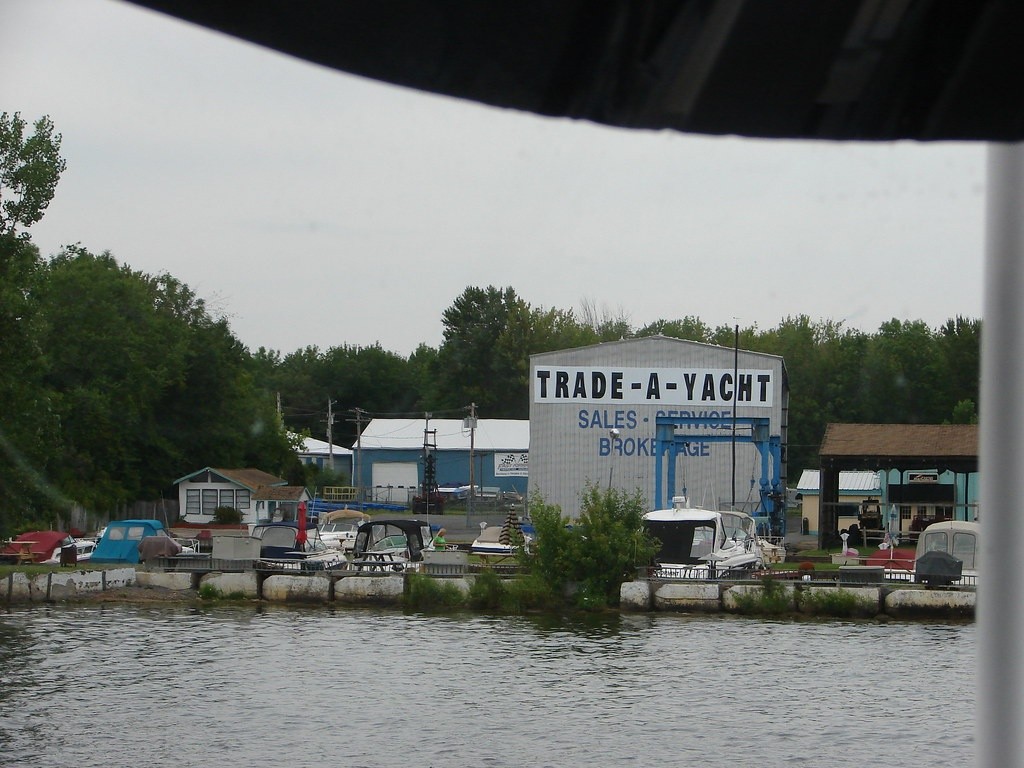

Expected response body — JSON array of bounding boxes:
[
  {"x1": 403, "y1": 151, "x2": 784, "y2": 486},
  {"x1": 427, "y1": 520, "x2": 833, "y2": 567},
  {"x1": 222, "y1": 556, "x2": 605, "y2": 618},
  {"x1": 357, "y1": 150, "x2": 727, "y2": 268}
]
[{"x1": 433, "y1": 529, "x2": 451, "y2": 551}]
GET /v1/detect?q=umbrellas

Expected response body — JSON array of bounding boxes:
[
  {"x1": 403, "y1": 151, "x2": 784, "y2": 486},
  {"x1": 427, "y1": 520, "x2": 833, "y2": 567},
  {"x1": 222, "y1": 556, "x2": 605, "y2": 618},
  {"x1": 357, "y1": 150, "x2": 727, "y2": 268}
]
[
  {"x1": 297, "y1": 500, "x2": 307, "y2": 552},
  {"x1": 887, "y1": 504, "x2": 899, "y2": 559},
  {"x1": 498, "y1": 504, "x2": 525, "y2": 554}
]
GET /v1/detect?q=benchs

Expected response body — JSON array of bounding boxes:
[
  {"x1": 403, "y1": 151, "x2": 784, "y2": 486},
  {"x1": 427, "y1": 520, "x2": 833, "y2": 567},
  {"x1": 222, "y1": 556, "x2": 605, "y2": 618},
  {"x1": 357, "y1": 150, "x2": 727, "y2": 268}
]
[
  {"x1": 351, "y1": 560, "x2": 407, "y2": 572},
  {"x1": 0, "y1": 552, "x2": 46, "y2": 559}
]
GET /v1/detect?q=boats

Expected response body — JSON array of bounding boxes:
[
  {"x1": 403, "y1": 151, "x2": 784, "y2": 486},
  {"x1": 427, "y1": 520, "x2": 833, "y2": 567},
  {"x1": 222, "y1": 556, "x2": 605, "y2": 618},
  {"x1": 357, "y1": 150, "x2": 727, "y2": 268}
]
[
  {"x1": 0, "y1": 486, "x2": 585, "y2": 573},
  {"x1": 909, "y1": 520, "x2": 980, "y2": 585},
  {"x1": 637, "y1": 496, "x2": 781, "y2": 581}
]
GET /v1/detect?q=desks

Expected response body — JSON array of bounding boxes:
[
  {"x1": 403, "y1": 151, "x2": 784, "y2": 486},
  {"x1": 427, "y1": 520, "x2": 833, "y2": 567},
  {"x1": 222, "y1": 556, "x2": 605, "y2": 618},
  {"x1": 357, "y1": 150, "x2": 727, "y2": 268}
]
[
  {"x1": 5, "y1": 541, "x2": 40, "y2": 565},
  {"x1": 334, "y1": 537, "x2": 356, "y2": 547},
  {"x1": 356, "y1": 551, "x2": 399, "y2": 576}
]
